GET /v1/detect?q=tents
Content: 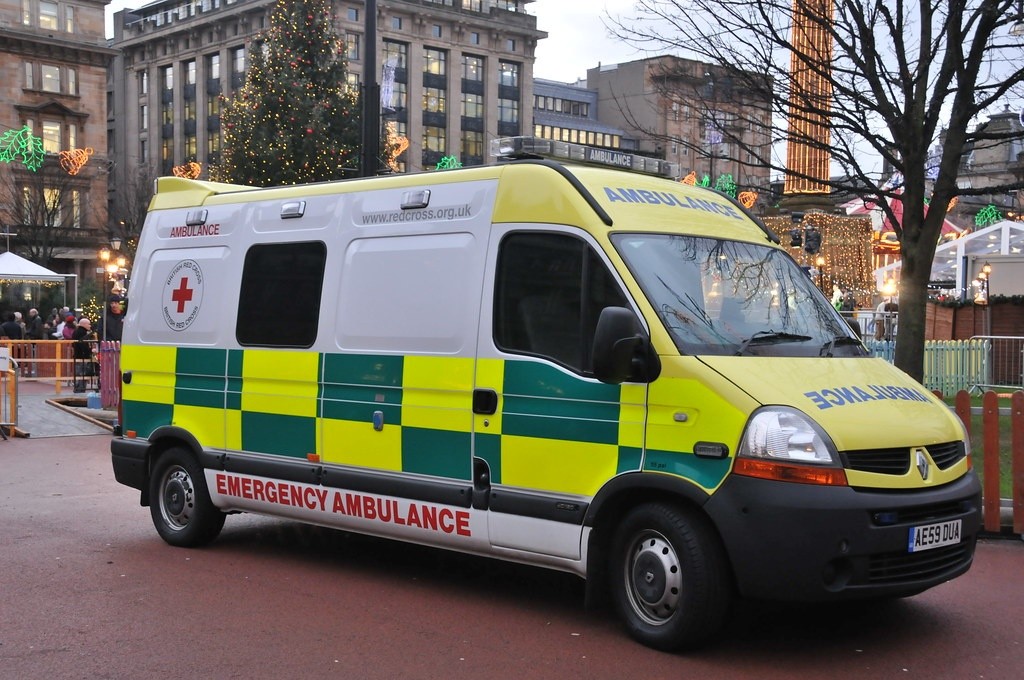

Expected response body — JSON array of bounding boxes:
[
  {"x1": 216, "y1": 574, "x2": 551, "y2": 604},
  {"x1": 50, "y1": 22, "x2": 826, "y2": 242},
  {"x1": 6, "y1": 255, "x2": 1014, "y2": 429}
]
[
  {"x1": 0, "y1": 251, "x2": 79, "y2": 312},
  {"x1": 827, "y1": 170, "x2": 966, "y2": 238}
]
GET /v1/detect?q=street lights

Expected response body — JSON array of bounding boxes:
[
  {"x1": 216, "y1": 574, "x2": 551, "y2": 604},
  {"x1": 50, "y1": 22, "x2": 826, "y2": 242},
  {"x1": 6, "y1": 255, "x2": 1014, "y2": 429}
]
[
  {"x1": 983, "y1": 261, "x2": 991, "y2": 336},
  {"x1": 99, "y1": 236, "x2": 125, "y2": 341},
  {"x1": 978, "y1": 268, "x2": 987, "y2": 336}
]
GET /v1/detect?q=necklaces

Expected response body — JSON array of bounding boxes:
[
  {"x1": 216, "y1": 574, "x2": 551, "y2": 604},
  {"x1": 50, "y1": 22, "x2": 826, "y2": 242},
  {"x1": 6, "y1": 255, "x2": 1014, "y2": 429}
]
[{"x1": 0, "y1": 293, "x2": 127, "y2": 372}]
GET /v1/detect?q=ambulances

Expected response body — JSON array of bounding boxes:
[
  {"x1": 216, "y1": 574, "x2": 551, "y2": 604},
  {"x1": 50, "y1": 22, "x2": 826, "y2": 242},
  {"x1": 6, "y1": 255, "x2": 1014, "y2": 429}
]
[{"x1": 109, "y1": 135, "x2": 981, "y2": 653}]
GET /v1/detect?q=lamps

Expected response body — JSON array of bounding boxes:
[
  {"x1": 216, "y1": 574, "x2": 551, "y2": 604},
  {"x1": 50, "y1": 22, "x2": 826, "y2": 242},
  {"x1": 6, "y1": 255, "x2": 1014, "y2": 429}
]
[{"x1": 973, "y1": 263, "x2": 991, "y2": 305}]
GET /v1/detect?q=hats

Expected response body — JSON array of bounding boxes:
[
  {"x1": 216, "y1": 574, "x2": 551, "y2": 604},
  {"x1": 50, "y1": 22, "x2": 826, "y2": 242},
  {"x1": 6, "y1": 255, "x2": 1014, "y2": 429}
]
[{"x1": 66, "y1": 316, "x2": 75, "y2": 321}]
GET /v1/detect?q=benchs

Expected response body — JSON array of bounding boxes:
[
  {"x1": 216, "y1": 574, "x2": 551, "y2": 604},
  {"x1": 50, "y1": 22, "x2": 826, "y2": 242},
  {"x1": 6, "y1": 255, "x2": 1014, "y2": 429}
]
[{"x1": 0, "y1": 358, "x2": 100, "y2": 380}]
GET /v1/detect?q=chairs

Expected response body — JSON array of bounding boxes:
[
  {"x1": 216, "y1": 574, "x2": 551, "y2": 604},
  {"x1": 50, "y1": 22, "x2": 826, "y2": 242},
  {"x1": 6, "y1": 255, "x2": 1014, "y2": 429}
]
[{"x1": 509, "y1": 279, "x2": 605, "y2": 374}]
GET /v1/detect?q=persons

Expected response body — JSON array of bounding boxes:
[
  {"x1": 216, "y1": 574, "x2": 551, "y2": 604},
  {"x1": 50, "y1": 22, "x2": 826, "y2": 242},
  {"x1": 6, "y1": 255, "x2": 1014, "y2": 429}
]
[
  {"x1": 875, "y1": 299, "x2": 889, "y2": 341},
  {"x1": 830, "y1": 283, "x2": 844, "y2": 306},
  {"x1": 841, "y1": 288, "x2": 857, "y2": 317}
]
